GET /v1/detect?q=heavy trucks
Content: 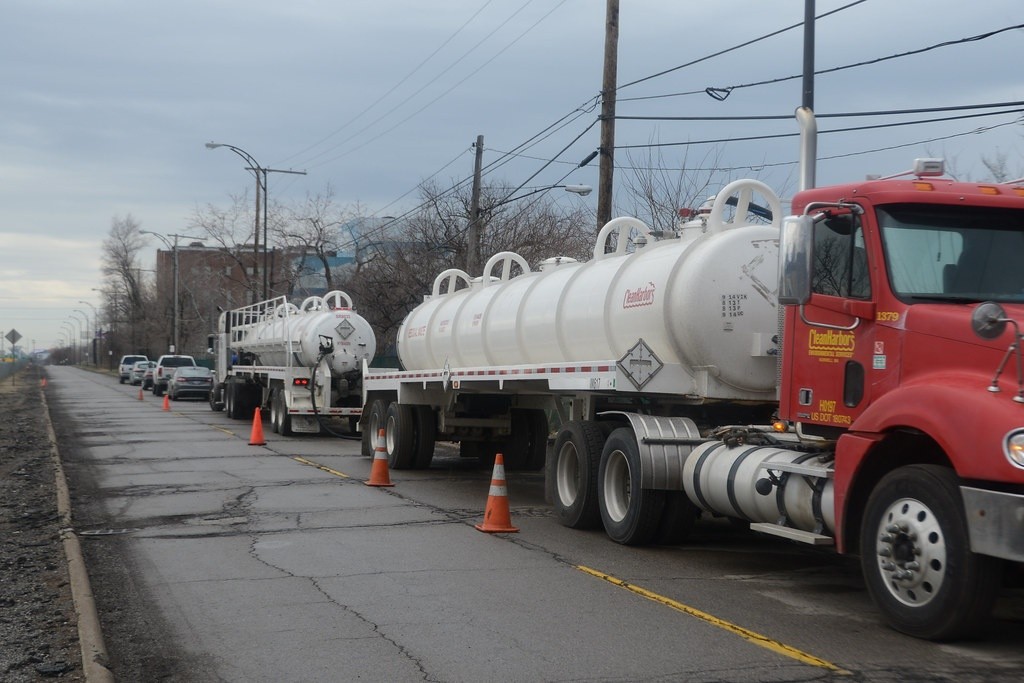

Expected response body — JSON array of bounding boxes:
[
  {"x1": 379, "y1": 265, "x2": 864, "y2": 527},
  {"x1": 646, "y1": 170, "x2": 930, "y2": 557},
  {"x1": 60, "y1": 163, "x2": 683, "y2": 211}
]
[
  {"x1": 357, "y1": 105, "x2": 1024, "y2": 642},
  {"x1": 207, "y1": 290, "x2": 400, "y2": 441}
]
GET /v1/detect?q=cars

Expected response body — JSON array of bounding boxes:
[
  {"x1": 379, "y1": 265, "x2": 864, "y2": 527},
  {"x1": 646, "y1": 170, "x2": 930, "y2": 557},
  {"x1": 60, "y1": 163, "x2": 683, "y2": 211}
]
[
  {"x1": 141, "y1": 368, "x2": 155, "y2": 390},
  {"x1": 129, "y1": 361, "x2": 157, "y2": 386},
  {"x1": 167, "y1": 367, "x2": 214, "y2": 402}
]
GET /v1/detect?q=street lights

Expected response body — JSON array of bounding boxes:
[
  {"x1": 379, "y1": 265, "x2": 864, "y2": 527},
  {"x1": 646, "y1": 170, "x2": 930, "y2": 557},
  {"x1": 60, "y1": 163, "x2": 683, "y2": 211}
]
[
  {"x1": 58, "y1": 289, "x2": 114, "y2": 372},
  {"x1": 464, "y1": 184, "x2": 593, "y2": 284},
  {"x1": 138, "y1": 228, "x2": 179, "y2": 355},
  {"x1": 206, "y1": 141, "x2": 268, "y2": 312}
]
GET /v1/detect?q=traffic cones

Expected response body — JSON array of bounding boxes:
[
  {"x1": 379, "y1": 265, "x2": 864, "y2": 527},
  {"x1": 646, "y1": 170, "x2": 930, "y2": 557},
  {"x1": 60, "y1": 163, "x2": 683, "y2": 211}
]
[
  {"x1": 474, "y1": 453, "x2": 521, "y2": 533},
  {"x1": 362, "y1": 429, "x2": 396, "y2": 486},
  {"x1": 137, "y1": 386, "x2": 143, "y2": 400},
  {"x1": 248, "y1": 408, "x2": 267, "y2": 445},
  {"x1": 164, "y1": 395, "x2": 170, "y2": 410}
]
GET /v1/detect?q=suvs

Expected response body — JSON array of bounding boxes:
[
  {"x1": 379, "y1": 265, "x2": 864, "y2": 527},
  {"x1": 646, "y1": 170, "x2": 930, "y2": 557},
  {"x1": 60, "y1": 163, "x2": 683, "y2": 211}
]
[
  {"x1": 119, "y1": 356, "x2": 148, "y2": 384},
  {"x1": 153, "y1": 354, "x2": 197, "y2": 396}
]
